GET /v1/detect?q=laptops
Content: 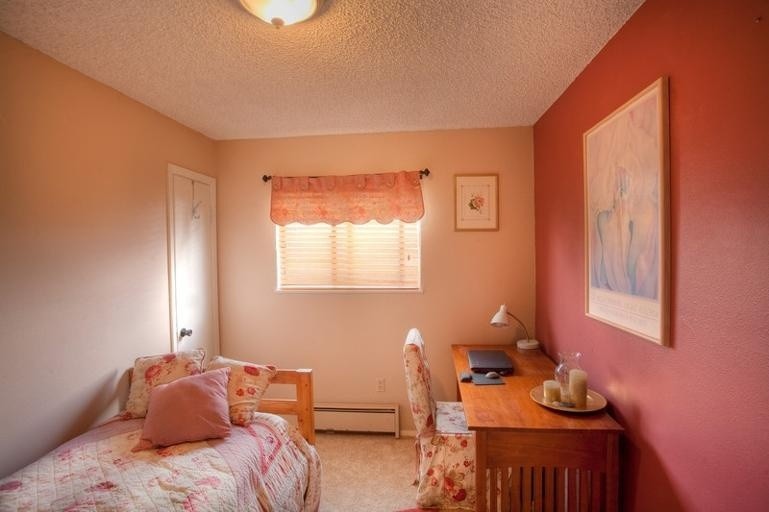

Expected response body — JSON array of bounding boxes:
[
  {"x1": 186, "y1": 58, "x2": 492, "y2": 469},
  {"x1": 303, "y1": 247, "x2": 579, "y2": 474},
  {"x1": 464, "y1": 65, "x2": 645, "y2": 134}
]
[{"x1": 468, "y1": 350, "x2": 514, "y2": 375}]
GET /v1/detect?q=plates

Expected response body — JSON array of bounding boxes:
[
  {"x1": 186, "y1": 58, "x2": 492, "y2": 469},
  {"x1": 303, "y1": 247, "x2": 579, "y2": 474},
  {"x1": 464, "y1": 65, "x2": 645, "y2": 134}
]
[{"x1": 529, "y1": 383, "x2": 608, "y2": 413}]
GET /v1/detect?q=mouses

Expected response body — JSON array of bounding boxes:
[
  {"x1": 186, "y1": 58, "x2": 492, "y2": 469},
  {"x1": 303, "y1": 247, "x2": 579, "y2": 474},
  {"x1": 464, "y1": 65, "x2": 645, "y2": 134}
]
[{"x1": 486, "y1": 372, "x2": 499, "y2": 379}]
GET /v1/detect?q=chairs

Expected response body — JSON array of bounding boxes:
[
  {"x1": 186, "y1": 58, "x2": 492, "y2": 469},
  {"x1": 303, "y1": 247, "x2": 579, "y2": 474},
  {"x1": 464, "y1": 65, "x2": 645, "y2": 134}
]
[{"x1": 402, "y1": 327, "x2": 512, "y2": 510}]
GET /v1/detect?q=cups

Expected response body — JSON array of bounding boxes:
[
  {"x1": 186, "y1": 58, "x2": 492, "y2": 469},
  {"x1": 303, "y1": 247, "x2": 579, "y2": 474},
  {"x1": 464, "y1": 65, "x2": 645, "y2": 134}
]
[
  {"x1": 543, "y1": 379, "x2": 562, "y2": 403},
  {"x1": 568, "y1": 368, "x2": 588, "y2": 409}
]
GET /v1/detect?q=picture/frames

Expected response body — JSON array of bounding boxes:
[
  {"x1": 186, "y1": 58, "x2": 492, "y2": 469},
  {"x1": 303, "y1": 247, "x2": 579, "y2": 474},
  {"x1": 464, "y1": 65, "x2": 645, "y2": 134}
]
[
  {"x1": 581, "y1": 76, "x2": 671, "y2": 347},
  {"x1": 452, "y1": 173, "x2": 501, "y2": 233}
]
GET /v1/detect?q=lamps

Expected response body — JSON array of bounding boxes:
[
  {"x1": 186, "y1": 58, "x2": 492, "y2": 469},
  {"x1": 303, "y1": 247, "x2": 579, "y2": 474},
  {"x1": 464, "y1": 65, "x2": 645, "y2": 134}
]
[
  {"x1": 239, "y1": 1, "x2": 318, "y2": 28},
  {"x1": 490, "y1": 303, "x2": 539, "y2": 350}
]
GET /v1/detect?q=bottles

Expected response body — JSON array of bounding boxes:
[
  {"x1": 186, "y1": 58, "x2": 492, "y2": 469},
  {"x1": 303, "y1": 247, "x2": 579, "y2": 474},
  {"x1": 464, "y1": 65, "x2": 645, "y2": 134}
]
[{"x1": 554, "y1": 350, "x2": 583, "y2": 405}]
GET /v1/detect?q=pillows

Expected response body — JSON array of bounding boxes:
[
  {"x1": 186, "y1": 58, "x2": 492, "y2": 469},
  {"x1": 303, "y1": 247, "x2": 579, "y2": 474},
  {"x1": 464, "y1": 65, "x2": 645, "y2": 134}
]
[{"x1": 122, "y1": 349, "x2": 276, "y2": 453}]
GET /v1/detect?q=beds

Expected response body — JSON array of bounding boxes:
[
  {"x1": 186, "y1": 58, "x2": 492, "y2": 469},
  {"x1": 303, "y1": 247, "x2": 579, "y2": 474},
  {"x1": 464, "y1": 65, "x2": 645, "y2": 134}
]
[{"x1": 0, "y1": 367, "x2": 316, "y2": 511}]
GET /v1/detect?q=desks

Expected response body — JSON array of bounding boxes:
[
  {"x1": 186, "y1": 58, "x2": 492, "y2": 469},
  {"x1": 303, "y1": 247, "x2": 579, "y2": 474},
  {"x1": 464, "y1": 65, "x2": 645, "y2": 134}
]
[{"x1": 450, "y1": 343, "x2": 627, "y2": 512}]
